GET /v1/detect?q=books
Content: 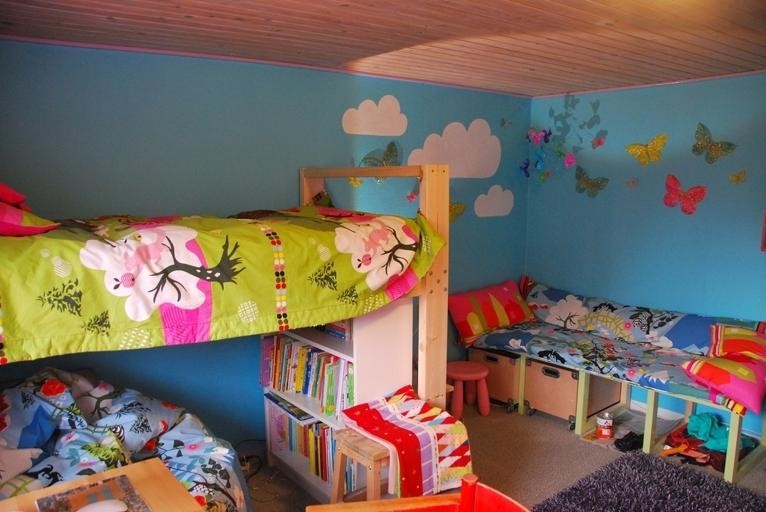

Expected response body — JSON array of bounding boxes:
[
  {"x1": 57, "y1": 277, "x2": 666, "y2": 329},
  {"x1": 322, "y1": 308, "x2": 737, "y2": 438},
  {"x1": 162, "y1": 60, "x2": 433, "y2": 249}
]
[{"x1": 260, "y1": 319, "x2": 355, "y2": 496}]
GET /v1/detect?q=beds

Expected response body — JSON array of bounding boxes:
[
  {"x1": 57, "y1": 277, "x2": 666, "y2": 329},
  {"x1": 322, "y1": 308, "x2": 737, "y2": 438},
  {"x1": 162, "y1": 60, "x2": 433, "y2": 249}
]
[
  {"x1": 1, "y1": 162, "x2": 445, "y2": 512},
  {"x1": 465, "y1": 275, "x2": 766, "y2": 485}
]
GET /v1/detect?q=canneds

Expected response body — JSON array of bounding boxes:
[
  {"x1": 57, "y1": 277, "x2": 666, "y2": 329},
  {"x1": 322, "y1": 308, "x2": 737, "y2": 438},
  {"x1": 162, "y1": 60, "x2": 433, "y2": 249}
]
[{"x1": 596, "y1": 412, "x2": 614, "y2": 439}]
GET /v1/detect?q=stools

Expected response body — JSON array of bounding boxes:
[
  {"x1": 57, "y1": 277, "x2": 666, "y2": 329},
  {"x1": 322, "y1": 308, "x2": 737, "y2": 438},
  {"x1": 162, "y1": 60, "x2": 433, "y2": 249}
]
[
  {"x1": 329, "y1": 428, "x2": 390, "y2": 504},
  {"x1": 445, "y1": 359, "x2": 491, "y2": 420}
]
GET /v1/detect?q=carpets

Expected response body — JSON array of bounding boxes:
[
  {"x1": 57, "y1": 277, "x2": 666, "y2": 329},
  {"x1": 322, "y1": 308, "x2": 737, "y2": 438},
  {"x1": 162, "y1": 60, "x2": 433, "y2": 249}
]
[{"x1": 529, "y1": 450, "x2": 766, "y2": 511}]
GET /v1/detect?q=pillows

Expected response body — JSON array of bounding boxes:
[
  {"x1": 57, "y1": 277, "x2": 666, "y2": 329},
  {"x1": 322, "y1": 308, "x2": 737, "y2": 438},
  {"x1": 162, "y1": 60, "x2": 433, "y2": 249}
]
[
  {"x1": 680, "y1": 323, "x2": 766, "y2": 415},
  {"x1": 448, "y1": 278, "x2": 538, "y2": 350}
]
